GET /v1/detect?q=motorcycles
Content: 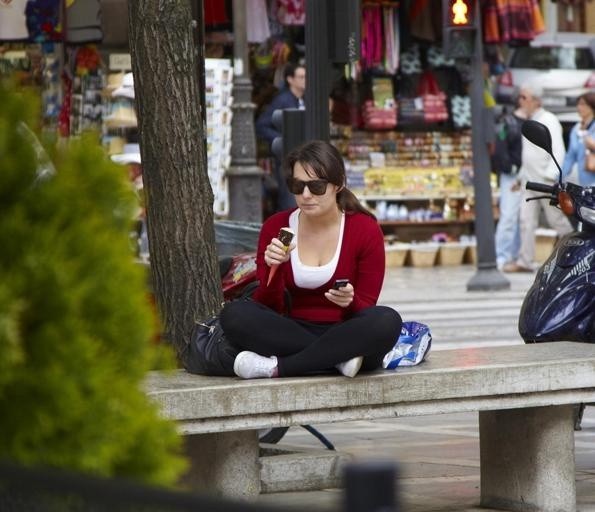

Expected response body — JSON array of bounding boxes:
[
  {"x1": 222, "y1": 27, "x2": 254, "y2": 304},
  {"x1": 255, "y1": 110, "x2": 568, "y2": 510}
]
[{"x1": 518, "y1": 121, "x2": 594, "y2": 343}]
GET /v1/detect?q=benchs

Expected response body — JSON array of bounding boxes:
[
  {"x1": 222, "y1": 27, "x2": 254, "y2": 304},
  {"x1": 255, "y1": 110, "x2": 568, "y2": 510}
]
[{"x1": 143, "y1": 340, "x2": 595, "y2": 512}]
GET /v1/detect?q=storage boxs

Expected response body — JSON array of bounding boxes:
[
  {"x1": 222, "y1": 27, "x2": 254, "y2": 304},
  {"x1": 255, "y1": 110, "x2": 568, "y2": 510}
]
[
  {"x1": 409, "y1": 241, "x2": 437, "y2": 265},
  {"x1": 534, "y1": 227, "x2": 559, "y2": 263},
  {"x1": 439, "y1": 242, "x2": 467, "y2": 265},
  {"x1": 383, "y1": 243, "x2": 411, "y2": 266},
  {"x1": 466, "y1": 241, "x2": 478, "y2": 265}
]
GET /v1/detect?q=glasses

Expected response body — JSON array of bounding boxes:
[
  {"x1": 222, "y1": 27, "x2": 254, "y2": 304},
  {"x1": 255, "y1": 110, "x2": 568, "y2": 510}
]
[{"x1": 287, "y1": 177, "x2": 328, "y2": 196}]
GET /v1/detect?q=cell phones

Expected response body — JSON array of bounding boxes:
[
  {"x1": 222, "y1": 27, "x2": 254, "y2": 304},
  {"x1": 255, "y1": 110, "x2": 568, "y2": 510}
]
[{"x1": 333, "y1": 279, "x2": 349, "y2": 290}]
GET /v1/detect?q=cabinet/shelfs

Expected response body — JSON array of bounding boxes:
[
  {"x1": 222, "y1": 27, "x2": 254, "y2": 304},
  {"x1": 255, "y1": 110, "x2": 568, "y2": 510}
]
[{"x1": 335, "y1": 130, "x2": 475, "y2": 240}]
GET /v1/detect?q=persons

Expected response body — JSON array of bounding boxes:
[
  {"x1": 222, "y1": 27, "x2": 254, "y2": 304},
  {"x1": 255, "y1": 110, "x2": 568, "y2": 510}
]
[
  {"x1": 256, "y1": 62, "x2": 334, "y2": 211},
  {"x1": 220, "y1": 139, "x2": 403, "y2": 379},
  {"x1": 505, "y1": 80, "x2": 577, "y2": 273},
  {"x1": 488, "y1": 82, "x2": 528, "y2": 271},
  {"x1": 555, "y1": 91, "x2": 595, "y2": 188}
]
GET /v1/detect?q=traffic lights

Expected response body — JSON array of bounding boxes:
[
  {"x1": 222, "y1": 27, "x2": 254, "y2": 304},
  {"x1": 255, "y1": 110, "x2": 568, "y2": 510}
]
[{"x1": 442, "y1": 0, "x2": 479, "y2": 60}]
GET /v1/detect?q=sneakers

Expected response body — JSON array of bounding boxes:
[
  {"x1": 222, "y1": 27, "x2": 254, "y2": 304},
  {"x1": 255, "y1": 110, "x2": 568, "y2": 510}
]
[
  {"x1": 232, "y1": 349, "x2": 280, "y2": 380},
  {"x1": 334, "y1": 355, "x2": 365, "y2": 378},
  {"x1": 503, "y1": 263, "x2": 534, "y2": 273}
]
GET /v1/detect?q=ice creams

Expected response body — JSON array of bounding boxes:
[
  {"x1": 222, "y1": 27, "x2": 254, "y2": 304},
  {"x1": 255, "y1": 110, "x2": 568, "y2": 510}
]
[{"x1": 267, "y1": 226, "x2": 295, "y2": 284}]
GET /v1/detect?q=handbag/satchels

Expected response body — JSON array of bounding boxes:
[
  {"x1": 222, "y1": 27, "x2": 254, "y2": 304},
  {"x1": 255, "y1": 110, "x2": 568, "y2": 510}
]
[{"x1": 585, "y1": 148, "x2": 595, "y2": 173}]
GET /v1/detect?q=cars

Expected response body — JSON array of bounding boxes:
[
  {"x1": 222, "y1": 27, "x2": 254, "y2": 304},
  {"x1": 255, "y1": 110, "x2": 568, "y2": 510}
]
[{"x1": 496, "y1": 32, "x2": 595, "y2": 107}]
